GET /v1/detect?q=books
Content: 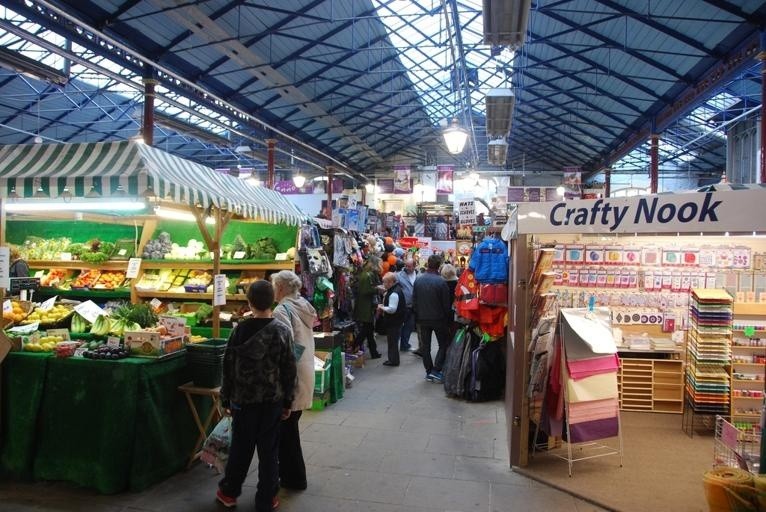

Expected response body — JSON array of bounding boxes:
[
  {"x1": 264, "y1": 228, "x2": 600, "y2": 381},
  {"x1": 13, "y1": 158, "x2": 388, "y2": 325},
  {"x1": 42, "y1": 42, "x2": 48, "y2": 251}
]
[
  {"x1": 531, "y1": 271, "x2": 557, "y2": 306},
  {"x1": 650, "y1": 338, "x2": 676, "y2": 350},
  {"x1": 531, "y1": 332, "x2": 553, "y2": 373},
  {"x1": 630, "y1": 335, "x2": 651, "y2": 350},
  {"x1": 529, "y1": 316, "x2": 557, "y2": 352},
  {"x1": 531, "y1": 249, "x2": 553, "y2": 285},
  {"x1": 531, "y1": 294, "x2": 555, "y2": 327},
  {"x1": 528, "y1": 352, "x2": 552, "y2": 395}
]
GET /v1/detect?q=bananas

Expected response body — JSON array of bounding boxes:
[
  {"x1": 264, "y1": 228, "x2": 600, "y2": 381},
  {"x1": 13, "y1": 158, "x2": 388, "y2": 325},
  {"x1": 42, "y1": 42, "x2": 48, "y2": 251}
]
[
  {"x1": 89, "y1": 314, "x2": 141, "y2": 335},
  {"x1": 70, "y1": 313, "x2": 84, "y2": 333}
]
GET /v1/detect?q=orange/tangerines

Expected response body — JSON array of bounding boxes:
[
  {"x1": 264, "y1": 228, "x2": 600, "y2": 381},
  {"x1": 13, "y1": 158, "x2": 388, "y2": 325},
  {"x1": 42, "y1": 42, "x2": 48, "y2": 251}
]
[{"x1": 13, "y1": 307, "x2": 21, "y2": 314}]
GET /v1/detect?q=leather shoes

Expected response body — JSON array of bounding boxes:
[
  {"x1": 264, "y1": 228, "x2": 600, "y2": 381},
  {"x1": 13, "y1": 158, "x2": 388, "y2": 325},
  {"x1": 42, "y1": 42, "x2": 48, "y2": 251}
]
[
  {"x1": 372, "y1": 353, "x2": 382, "y2": 358},
  {"x1": 385, "y1": 361, "x2": 389, "y2": 362},
  {"x1": 383, "y1": 362, "x2": 398, "y2": 366}
]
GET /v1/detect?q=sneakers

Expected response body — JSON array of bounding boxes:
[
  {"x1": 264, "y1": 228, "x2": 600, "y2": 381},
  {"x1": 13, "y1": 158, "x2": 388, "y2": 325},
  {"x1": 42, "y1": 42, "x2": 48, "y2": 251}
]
[
  {"x1": 217, "y1": 488, "x2": 236, "y2": 507},
  {"x1": 271, "y1": 497, "x2": 279, "y2": 509},
  {"x1": 429, "y1": 370, "x2": 443, "y2": 379},
  {"x1": 425, "y1": 373, "x2": 433, "y2": 381}
]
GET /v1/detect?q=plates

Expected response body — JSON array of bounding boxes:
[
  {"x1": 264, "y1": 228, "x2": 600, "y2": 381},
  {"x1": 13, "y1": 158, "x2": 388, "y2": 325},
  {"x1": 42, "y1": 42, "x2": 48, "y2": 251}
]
[
  {"x1": 109, "y1": 240, "x2": 135, "y2": 261},
  {"x1": 88, "y1": 270, "x2": 126, "y2": 290},
  {"x1": 688, "y1": 329, "x2": 732, "y2": 367},
  {"x1": 690, "y1": 288, "x2": 733, "y2": 335},
  {"x1": 71, "y1": 287, "x2": 89, "y2": 291},
  {"x1": 686, "y1": 363, "x2": 730, "y2": 413}
]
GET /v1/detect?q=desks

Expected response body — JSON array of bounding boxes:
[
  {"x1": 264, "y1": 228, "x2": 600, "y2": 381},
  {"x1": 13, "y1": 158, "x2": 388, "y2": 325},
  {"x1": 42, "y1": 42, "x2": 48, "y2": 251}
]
[{"x1": 0, "y1": 334, "x2": 207, "y2": 494}]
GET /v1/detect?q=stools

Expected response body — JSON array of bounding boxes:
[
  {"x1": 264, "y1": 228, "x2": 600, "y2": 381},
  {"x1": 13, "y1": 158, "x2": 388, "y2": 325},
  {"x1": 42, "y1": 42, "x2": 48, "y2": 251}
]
[{"x1": 178, "y1": 381, "x2": 236, "y2": 476}]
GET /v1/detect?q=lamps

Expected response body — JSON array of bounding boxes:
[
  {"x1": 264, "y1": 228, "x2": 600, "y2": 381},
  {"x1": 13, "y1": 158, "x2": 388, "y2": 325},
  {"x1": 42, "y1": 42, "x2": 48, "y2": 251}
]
[
  {"x1": 292, "y1": 165, "x2": 305, "y2": 189},
  {"x1": 441, "y1": 86, "x2": 469, "y2": 154}
]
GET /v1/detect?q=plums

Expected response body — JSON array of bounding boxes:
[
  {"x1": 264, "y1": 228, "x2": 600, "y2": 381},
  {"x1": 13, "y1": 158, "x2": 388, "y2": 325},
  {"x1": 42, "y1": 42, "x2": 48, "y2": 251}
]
[{"x1": 82, "y1": 344, "x2": 129, "y2": 359}]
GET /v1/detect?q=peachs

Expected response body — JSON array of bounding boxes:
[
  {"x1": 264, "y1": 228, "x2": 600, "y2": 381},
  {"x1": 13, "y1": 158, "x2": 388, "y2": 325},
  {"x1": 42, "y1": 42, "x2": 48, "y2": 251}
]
[{"x1": 99, "y1": 272, "x2": 123, "y2": 288}]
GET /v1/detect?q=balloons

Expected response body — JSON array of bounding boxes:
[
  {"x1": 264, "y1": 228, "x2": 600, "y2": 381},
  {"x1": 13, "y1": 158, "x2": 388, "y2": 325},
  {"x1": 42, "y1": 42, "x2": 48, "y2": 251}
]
[
  {"x1": 386, "y1": 237, "x2": 393, "y2": 244},
  {"x1": 383, "y1": 262, "x2": 389, "y2": 272},
  {"x1": 394, "y1": 248, "x2": 403, "y2": 256},
  {"x1": 385, "y1": 245, "x2": 395, "y2": 252},
  {"x1": 388, "y1": 256, "x2": 396, "y2": 265}
]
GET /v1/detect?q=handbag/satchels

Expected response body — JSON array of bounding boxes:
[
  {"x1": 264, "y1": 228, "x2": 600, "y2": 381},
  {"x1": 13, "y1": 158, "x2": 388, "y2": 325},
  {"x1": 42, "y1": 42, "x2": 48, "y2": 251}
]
[
  {"x1": 282, "y1": 304, "x2": 305, "y2": 361},
  {"x1": 442, "y1": 328, "x2": 474, "y2": 396},
  {"x1": 470, "y1": 346, "x2": 502, "y2": 401}
]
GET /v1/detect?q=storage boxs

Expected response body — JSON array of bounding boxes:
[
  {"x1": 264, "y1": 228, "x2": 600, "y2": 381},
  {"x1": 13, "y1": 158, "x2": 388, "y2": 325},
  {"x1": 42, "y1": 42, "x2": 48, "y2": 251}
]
[{"x1": 315, "y1": 347, "x2": 333, "y2": 395}]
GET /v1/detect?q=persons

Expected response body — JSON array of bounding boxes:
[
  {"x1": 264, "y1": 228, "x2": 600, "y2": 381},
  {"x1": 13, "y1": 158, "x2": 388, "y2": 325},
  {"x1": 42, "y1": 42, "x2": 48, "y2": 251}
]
[
  {"x1": 378, "y1": 272, "x2": 406, "y2": 367},
  {"x1": 442, "y1": 264, "x2": 459, "y2": 293},
  {"x1": 217, "y1": 280, "x2": 297, "y2": 510},
  {"x1": 1, "y1": 243, "x2": 30, "y2": 277},
  {"x1": 270, "y1": 270, "x2": 317, "y2": 490},
  {"x1": 414, "y1": 258, "x2": 429, "y2": 272},
  {"x1": 413, "y1": 255, "x2": 452, "y2": 382},
  {"x1": 443, "y1": 252, "x2": 456, "y2": 263},
  {"x1": 396, "y1": 258, "x2": 421, "y2": 352},
  {"x1": 353, "y1": 257, "x2": 385, "y2": 359}
]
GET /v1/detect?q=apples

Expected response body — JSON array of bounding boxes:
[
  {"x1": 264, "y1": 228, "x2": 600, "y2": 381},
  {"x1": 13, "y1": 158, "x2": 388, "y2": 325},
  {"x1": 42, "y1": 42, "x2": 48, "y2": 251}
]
[{"x1": 145, "y1": 325, "x2": 172, "y2": 339}]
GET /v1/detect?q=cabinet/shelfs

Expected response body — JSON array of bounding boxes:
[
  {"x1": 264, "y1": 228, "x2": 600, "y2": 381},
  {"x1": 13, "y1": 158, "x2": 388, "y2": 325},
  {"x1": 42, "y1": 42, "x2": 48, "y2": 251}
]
[
  {"x1": 0, "y1": 207, "x2": 299, "y2": 346},
  {"x1": 729, "y1": 299, "x2": 765, "y2": 443}
]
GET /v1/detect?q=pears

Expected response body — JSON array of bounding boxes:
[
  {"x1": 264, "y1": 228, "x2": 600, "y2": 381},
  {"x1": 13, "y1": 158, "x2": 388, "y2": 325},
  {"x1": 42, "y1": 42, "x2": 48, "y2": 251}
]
[
  {"x1": 47, "y1": 336, "x2": 54, "y2": 341},
  {"x1": 38, "y1": 337, "x2": 47, "y2": 344},
  {"x1": 54, "y1": 336, "x2": 62, "y2": 342},
  {"x1": 24, "y1": 343, "x2": 33, "y2": 351},
  {"x1": 32, "y1": 344, "x2": 41, "y2": 352},
  {"x1": 21, "y1": 304, "x2": 68, "y2": 324},
  {"x1": 40, "y1": 341, "x2": 56, "y2": 351}
]
